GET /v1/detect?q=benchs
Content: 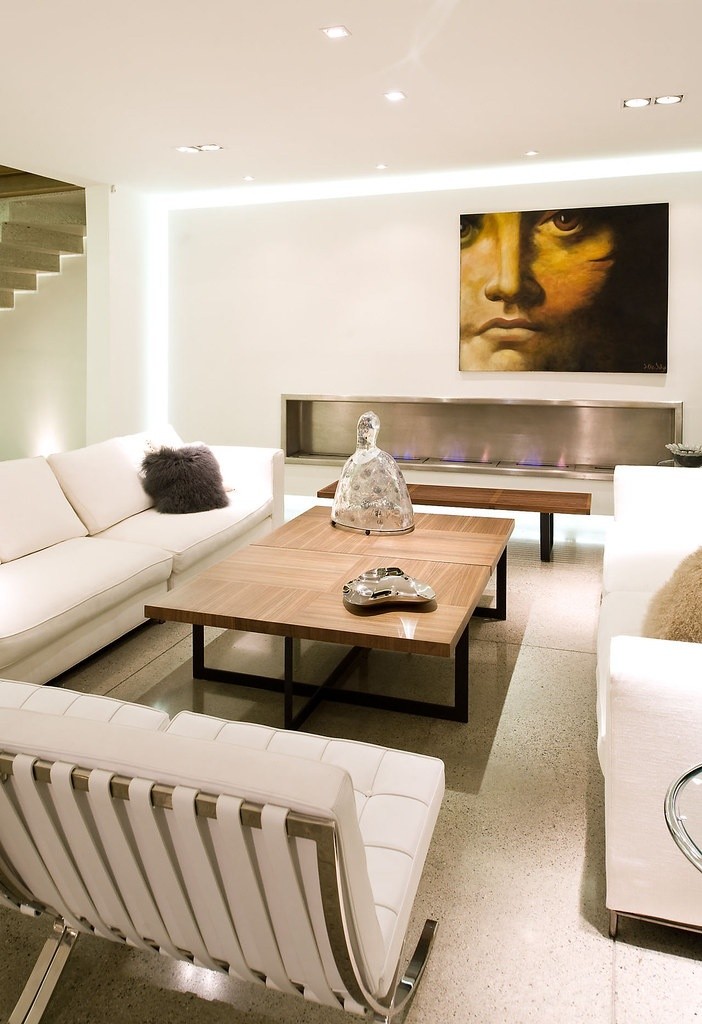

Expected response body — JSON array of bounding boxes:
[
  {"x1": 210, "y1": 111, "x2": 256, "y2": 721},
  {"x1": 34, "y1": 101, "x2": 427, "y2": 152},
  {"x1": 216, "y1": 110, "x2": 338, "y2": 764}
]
[
  {"x1": 317, "y1": 469, "x2": 592, "y2": 561},
  {"x1": 0, "y1": 678, "x2": 445, "y2": 1024}
]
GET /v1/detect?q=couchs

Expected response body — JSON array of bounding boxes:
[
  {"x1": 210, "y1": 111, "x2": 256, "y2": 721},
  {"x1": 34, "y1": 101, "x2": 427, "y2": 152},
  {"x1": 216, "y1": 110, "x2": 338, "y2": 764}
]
[
  {"x1": 596, "y1": 465, "x2": 702, "y2": 947},
  {"x1": 0, "y1": 433, "x2": 284, "y2": 685}
]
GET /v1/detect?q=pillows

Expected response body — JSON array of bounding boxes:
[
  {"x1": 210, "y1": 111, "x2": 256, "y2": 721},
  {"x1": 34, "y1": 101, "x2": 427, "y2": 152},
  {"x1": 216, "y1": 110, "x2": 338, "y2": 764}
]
[
  {"x1": 0, "y1": 433, "x2": 230, "y2": 564},
  {"x1": 642, "y1": 546, "x2": 702, "y2": 644}
]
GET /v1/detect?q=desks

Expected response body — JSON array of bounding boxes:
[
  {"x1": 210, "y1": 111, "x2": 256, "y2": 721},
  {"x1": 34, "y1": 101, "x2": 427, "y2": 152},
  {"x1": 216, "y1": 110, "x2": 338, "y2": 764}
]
[{"x1": 144, "y1": 505, "x2": 515, "y2": 730}]
